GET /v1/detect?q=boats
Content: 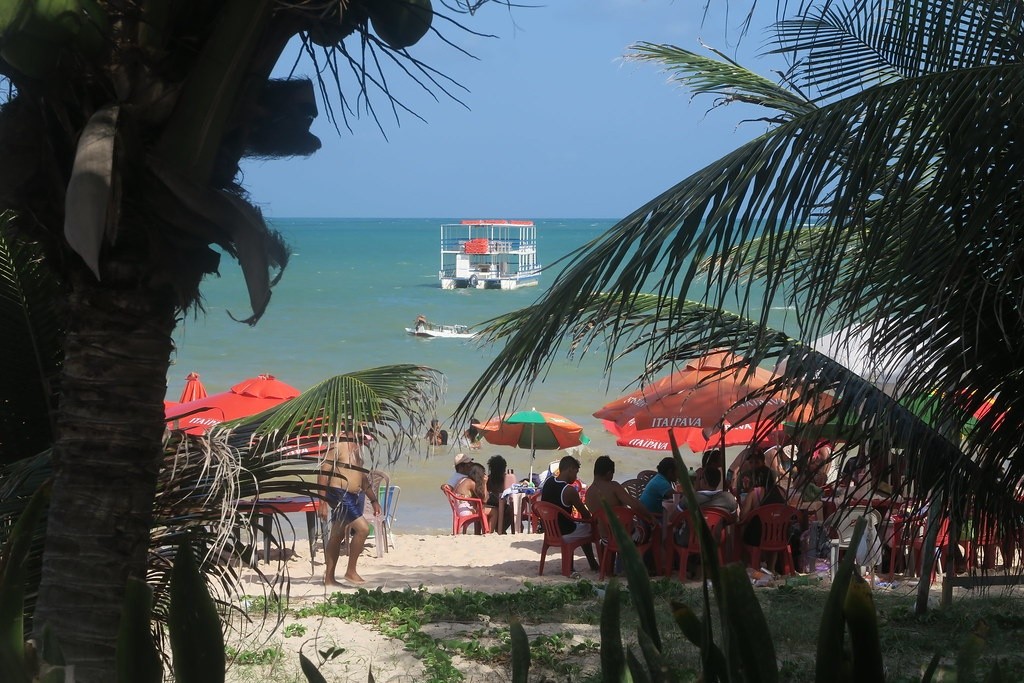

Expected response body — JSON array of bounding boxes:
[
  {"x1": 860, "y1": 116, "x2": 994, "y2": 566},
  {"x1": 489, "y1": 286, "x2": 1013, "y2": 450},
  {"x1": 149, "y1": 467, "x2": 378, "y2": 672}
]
[{"x1": 404, "y1": 315, "x2": 480, "y2": 340}]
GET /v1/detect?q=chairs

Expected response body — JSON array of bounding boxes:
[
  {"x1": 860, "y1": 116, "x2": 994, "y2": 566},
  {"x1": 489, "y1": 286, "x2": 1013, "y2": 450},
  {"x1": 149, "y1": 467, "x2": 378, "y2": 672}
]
[
  {"x1": 441, "y1": 483, "x2": 489, "y2": 535},
  {"x1": 821, "y1": 505, "x2": 881, "y2": 590},
  {"x1": 316, "y1": 471, "x2": 400, "y2": 558},
  {"x1": 889, "y1": 509, "x2": 1024, "y2": 580},
  {"x1": 531, "y1": 470, "x2": 803, "y2": 578}
]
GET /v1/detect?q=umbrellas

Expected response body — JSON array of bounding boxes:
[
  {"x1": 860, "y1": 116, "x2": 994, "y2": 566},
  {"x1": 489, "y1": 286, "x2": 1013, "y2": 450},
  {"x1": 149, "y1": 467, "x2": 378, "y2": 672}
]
[
  {"x1": 467, "y1": 408, "x2": 583, "y2": 488},
  {"x1": 167, "y1": 368, "x2": 375, "y2": 459},
  {"x1": 589, "y1": 341, "x2": 1023, "y2": 460}
]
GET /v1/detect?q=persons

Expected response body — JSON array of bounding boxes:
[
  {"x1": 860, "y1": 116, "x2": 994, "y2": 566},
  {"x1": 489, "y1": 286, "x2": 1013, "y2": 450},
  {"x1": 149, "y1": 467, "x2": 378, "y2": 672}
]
[
  {"x1": 449, "y1": 425, "x2": 1024, "y2": 594},
  {"x1": 314, "y1": 408, "x2": 378, "y2": 588},
  {"x1": 415, "y1": 315, "x2": 426, "y2": 332},
  {"x1": 465, "y1": 418, "x2": 483, "y2": 450},
  {"x1": 424, "y1": 420, "x2": 443, "y2": 446}
]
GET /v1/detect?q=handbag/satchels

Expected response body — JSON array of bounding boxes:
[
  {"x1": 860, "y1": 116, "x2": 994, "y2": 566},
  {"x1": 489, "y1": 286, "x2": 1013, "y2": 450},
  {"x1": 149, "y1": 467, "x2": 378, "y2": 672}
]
[{"x1": 877, "y1": 468, "x2": 893, "y2": 494}]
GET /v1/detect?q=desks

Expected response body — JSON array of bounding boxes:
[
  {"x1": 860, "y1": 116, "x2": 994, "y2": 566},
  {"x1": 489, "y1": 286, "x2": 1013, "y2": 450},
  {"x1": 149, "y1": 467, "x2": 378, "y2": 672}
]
[
  {"x1": 498, "y1": 493, "x2": 527, "y2": 535},
  {"x1": 197, "y1": 502, "x2": 331, "y2": 565},
  {"x1": 663, "y1": 496, "x2": 823, "y2": 569}
]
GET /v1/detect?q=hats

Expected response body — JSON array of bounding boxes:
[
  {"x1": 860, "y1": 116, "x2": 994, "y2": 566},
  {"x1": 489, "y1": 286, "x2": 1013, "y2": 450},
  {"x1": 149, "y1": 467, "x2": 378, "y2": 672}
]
[
  {"x1": 782, "y1": 444, "x2": 799, "y2": 461},
  {"x1": 454, "y1": 453, "x2": 474, "y2": 466}
]
